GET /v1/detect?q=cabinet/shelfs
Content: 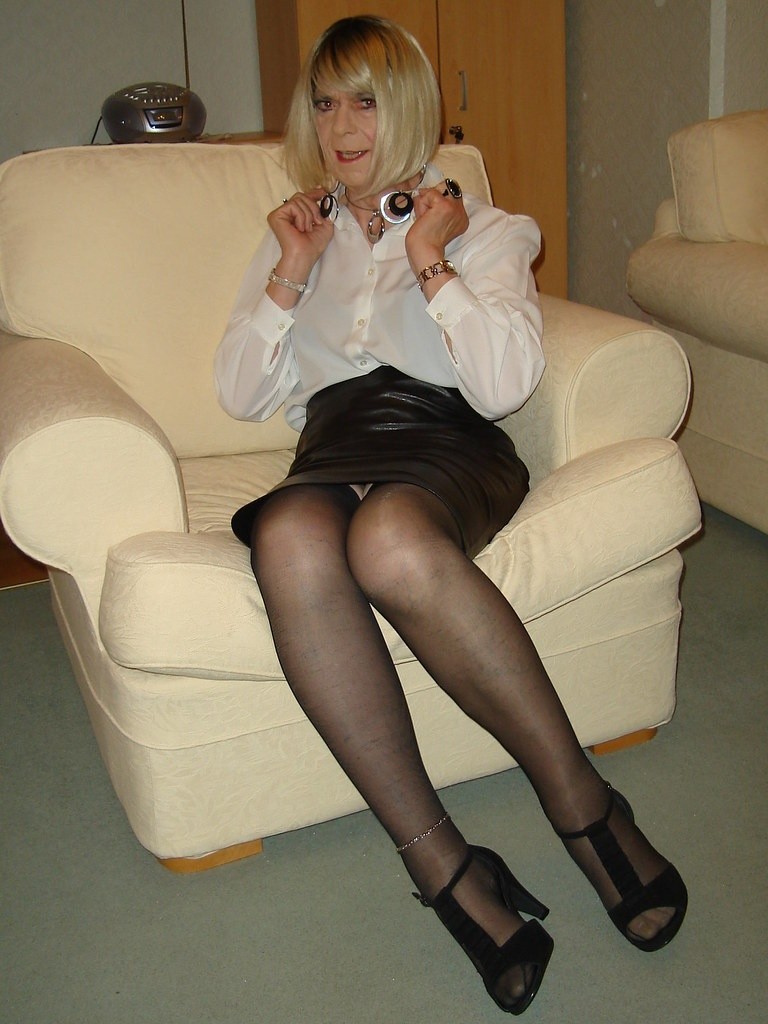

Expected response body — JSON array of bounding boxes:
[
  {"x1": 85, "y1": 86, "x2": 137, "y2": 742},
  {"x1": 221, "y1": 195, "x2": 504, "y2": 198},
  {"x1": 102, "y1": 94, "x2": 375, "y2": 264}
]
[{"x1": 254, "y1": 1, "x2": 567, "y2": 298}]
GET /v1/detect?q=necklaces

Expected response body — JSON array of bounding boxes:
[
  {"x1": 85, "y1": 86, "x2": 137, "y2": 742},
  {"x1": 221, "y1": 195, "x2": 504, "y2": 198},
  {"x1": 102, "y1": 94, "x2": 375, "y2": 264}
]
[{"x1": 344, "y1": 163, "x2": 427, "y2": 245}]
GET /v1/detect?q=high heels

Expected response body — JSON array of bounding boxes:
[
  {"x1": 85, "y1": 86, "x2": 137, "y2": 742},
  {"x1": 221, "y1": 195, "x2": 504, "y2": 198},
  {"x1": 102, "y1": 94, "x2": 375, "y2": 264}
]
[
  {"x1": 412, "y1": 844, "x2": 554, "y2": 1016},
  {"x1": 557, "y1": 778, "x2": 688, "y2": 951}
]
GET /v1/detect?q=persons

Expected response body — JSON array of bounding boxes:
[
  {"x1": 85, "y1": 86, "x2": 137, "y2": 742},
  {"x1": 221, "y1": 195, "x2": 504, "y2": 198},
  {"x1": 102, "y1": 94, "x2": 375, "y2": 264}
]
[{"x1": 209, "y1": 13, "x2": 689, "y2": 1017}]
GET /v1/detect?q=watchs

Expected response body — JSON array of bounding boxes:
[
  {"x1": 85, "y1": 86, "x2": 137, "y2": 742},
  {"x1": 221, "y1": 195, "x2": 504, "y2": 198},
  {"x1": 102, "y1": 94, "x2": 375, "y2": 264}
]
[
  {"x1": 267, "y1": 265, "x2": 307, "y2": 294},
  {"x1": 414, "y1": 259, "x2": 459, "y2": 293}
]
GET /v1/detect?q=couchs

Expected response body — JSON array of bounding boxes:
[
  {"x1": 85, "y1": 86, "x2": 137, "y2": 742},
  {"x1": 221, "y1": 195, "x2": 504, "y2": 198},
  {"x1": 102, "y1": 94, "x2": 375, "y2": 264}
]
[
  {"x1": 1, "y1": 145, "x2": 703, "y2": 877},
  {"x1": 628, "y1": 110, "x2": 767, "y2": 539}
]
